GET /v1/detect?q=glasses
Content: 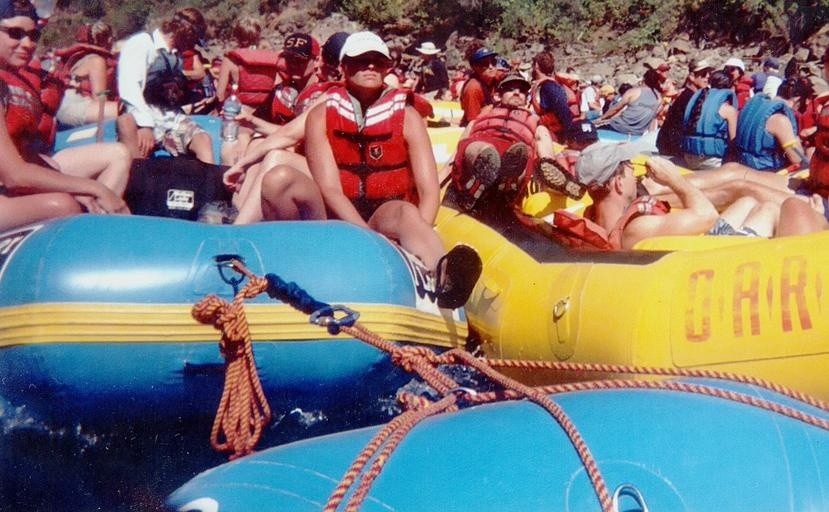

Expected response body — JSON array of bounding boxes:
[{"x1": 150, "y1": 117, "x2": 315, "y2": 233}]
[
  {"x1": 624, "y1": 159, "x2": 653, "y2": 181},
  {"x1": 0, "y1": 25, "x2": 41, "y2": 43}
]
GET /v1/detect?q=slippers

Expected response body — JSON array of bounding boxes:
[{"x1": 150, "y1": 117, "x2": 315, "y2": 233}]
[{"x1": 435, "y1": 246, "x2": 483, "y2": 309}]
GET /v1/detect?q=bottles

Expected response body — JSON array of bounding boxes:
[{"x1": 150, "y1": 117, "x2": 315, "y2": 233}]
[{"x1": 221, "y1": 84, "x2": 241, "y2": 141}]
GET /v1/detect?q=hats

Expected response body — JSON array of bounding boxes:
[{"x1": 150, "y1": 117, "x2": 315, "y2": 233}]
[
  {"x1": 694, "y1": 60, "x2": 716, "y2": 72},
  {"x1": 575, "y1": 139, "x2": 640, "y2": 191},
  {"x1": 598, "y1": 84, "x2": 618, "y2": 96},
  {"x1": 723, "y1": 57, "x2": 745, "y2": 72},
  {"x1": 468, "y1": 46, "x2": 498, "y2": 61},
  {"x1": 173, "y1": 15, "x2": 210, "y2": 53},
  {"x1": 763, "y1": 58, "x2": 780, "y2": 70},
  {"x1": 323, "y1": 32, "x2": 350, "y2": 60},
  {"x1": 338, "y1": 31, "x2": 393, "y2": 65},
  {"x1": 278, "y1": 31, "x2": 320, "y2": 61},
  {"x1": 498, "y1": 72, "x2": 531, "y2": 91},
  {"x1": 415, "y1": 41, "x2": 442, "y2": 55},
  {"x1": 0, "y1": 1, "x2": 38, "y2": 24}
]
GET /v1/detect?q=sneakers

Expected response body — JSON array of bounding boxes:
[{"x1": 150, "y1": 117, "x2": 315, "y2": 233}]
[
  {"x1": 456, "y1": 145, "x2": 501, "y2": 212},
  {"x1": 498, "y1": 141, "x2": 530, "y2": 201},
  {"x1": 537, "y1": 157, "x2": 586, "y2": 201}
]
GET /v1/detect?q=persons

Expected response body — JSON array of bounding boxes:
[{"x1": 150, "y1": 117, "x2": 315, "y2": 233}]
[
  {"x1": 259, "y1": 29, "x2": 484, "y2": 310},
  {"x1": 49, "y1": 6, "x2": 352, "y2": 224},
  {"x1": 411, "y1": 41, "x2": 450, "y2": 100},
  {"x1": 0, "y1": 0, "x2": 134, "y2": 232},
  {"x1": 448, "y1": 41, "x2": 829, "y2": 253}
]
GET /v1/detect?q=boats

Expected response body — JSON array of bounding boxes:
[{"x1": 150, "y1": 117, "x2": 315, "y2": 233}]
[
  {"x1": 409, "y1": 124, "x2": 823, "y2": 407},
  {"x1": 1, "y1": 113, "x2": 471, "y2": 421},
  {"x1": 147, "y1": 376, "x2": 827, "y2": 512}
]
